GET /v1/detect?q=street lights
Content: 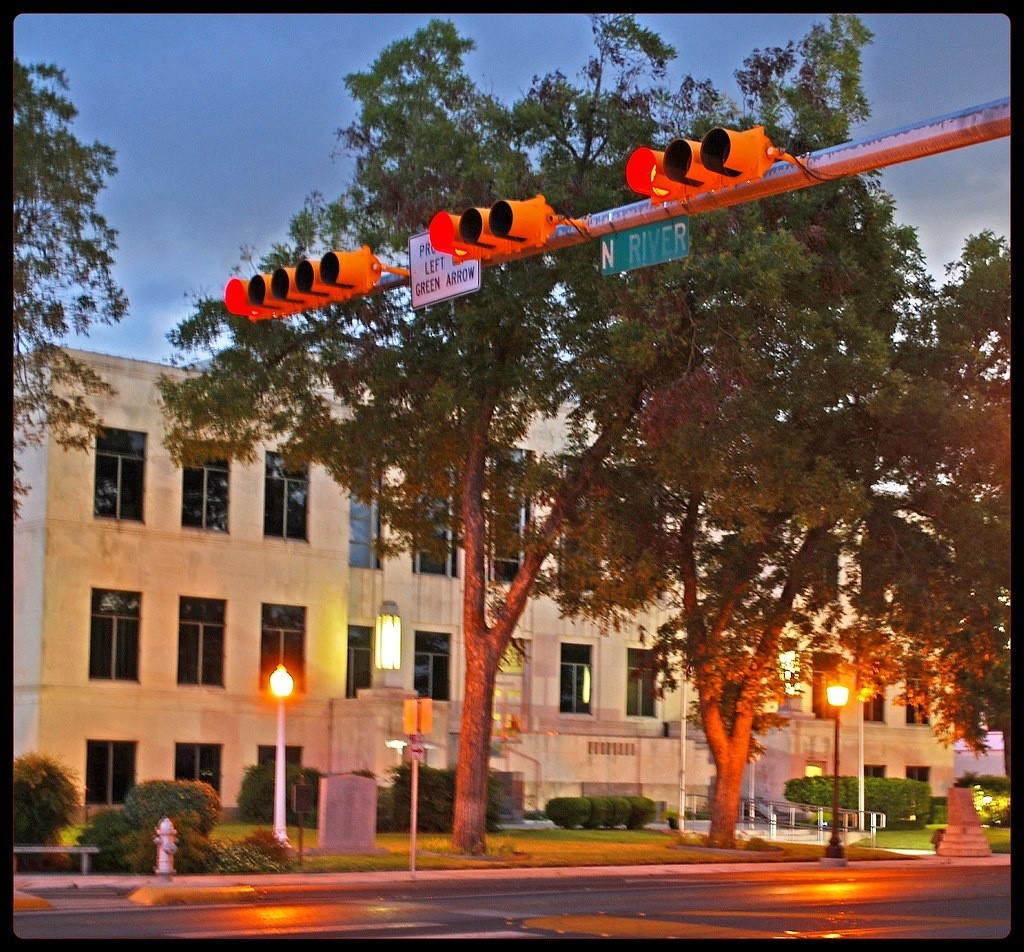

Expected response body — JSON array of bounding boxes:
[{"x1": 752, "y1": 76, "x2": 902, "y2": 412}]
[
  {"x1": 823, "y1": 685, "x2": 852, "y2": 869},
  {"x1": 270, "y1": 664, "x2": 298, "y2": 850}
]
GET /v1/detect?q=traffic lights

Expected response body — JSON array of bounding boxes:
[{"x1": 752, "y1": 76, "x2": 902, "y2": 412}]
[
  {"x1": 222, "y1": 245, "x2": 384, "y2": 322},
  {"x1": 427, "y1": 192, "x2": 556, "y2": 260},
  {"x1": 625, "y1": 128, "x2": 774, "y2": 207}
]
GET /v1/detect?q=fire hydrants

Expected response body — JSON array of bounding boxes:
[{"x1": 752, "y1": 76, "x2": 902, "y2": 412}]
[{"x1": 153, "y1": 818, "x2": 186, "y2": 886}]
